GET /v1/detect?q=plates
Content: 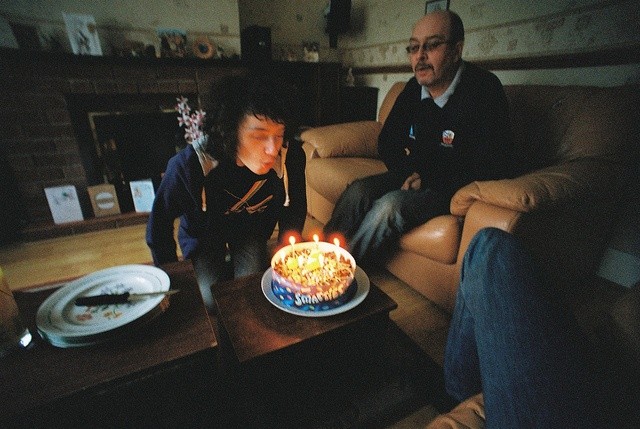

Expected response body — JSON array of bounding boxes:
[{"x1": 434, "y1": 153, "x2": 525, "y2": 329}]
[
  {"x1": 261, "y1": 264, "x2": 370, "y2": 318},
  {"x1": 34, "y1": 263, "x2": 171, "y2": 338}
]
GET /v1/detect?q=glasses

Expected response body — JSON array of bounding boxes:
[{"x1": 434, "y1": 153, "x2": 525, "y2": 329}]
[{"x1": 406, "y1": 41, "x2": 448, "y2": 54}]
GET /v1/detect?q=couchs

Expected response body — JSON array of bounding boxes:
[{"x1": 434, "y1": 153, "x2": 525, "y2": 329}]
[{"x1": 299, "y1": 80, "x2": 639, "y2": 316}]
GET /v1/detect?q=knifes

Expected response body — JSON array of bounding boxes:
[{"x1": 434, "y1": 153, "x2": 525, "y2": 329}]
[{"x1": 74, "y1": 288, "x2": 183, "y2": 305}]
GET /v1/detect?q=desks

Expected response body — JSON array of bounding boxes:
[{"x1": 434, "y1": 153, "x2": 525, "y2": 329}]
[
  {"x1": 2, "y1": 258, "x2": 218, "y2": 427},
  {"x1": 209, "y1": 264, "x2": 400, "y2": 413}
]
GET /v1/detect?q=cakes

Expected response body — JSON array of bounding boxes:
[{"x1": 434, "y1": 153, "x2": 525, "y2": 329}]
[{"x1": 271, "y1": 241, "x2": 357, "y2": 311}]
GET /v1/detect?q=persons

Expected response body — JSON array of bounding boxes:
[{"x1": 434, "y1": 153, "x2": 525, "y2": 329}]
[
  {"x1": 321, "y1": 8, "x2": 516, "y2": 273},
  {"x1": 144, "y1": 71, "x2": 308, "y2": 316},
  {"x1": 409, "y1": 227, "x2": 606, "y2": 429}
]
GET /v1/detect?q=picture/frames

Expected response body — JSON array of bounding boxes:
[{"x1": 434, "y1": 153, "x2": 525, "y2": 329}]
[{"x1": 424, "y1": 0, "x2": 450, "y2": 15}]
